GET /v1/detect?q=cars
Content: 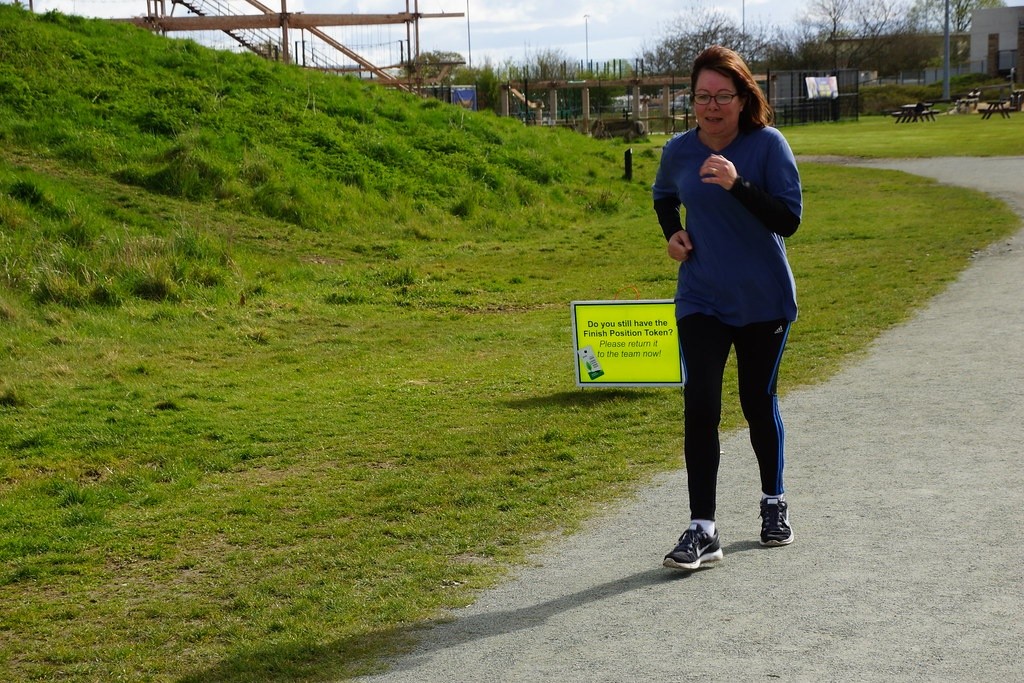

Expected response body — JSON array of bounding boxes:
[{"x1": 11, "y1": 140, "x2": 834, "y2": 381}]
[{"x1": 670, "y1": 94, "x2": 692, "y2": 110}]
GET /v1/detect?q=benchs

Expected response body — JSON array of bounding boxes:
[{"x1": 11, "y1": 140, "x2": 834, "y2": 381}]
[
  {"x1": 977, "y1": 105, "x2": 1015, "y2": 120},
  {"x1": 891, "y1": 110, "x2": 939, "y2": 123}
]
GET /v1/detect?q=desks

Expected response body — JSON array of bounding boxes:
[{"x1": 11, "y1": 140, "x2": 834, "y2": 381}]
[
  {"x1": 899, "y1": 102, "x2": 932, "y2": 124},
  {"x1": 986, "y1": 100, "x2": 1009, "y2": 119}
]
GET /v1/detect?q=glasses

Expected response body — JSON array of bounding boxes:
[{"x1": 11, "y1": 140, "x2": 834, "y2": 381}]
[{"x1": 692, "y1": 93, "x2": 742, "y2": 104}]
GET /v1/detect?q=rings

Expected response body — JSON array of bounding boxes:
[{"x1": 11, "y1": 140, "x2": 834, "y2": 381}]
[{"x1": 712, "y1": 168, "x2": 717, "y2": 174}]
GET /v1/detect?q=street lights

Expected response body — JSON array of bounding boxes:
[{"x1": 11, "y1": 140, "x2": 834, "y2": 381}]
[{"x1": 584, "y1": 15, "x2": 589, "y2": 69}]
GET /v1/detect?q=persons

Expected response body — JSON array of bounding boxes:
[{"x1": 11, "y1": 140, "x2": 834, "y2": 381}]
[{"x1": 652, "y1": 46, "x2": 803, "y2": 569}]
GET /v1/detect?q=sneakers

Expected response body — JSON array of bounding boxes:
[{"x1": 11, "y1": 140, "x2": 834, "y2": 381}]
[
  {"x1": 759, "y1": 497, "x2": 795, "y2": 546},
  {"x1": 663, "y1": 522, "x2": 724, "y2": 569}
]
